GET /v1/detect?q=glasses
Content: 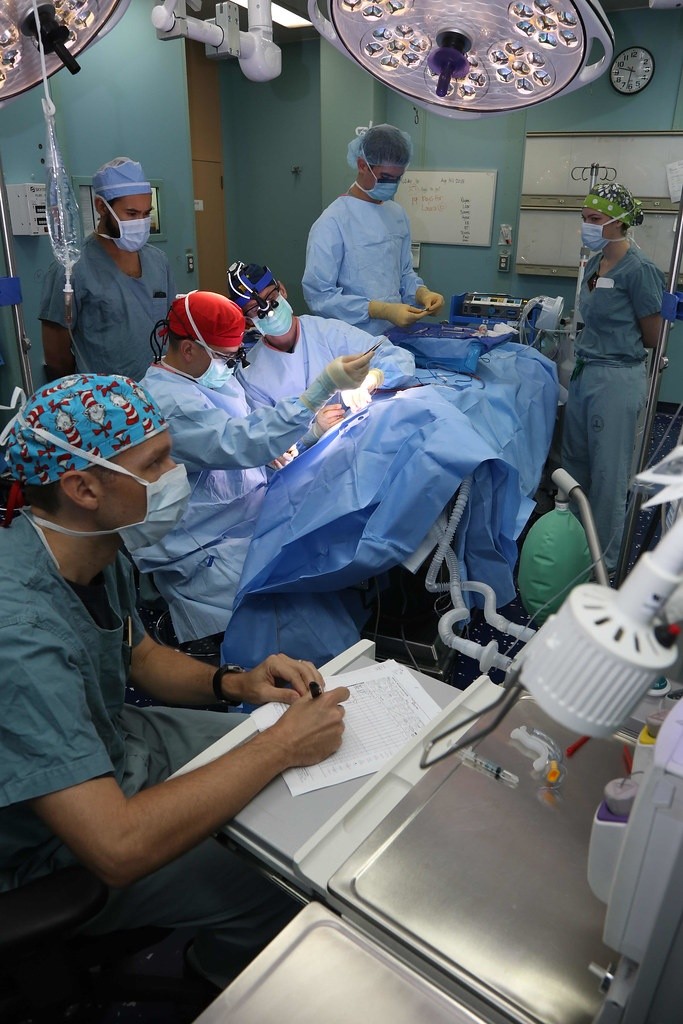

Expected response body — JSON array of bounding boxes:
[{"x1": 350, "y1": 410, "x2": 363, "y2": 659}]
[
  {"x1": 246, "y1": 285, "x2": 279, "y2": 318},
  {"x1": 195, "y1": 340, "x2": 241, "y2": 365}
]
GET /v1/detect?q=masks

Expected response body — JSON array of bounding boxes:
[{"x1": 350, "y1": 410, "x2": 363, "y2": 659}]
[
  {"x1": 581, "y1": 211, "x2": 626, "y2": 252},
  {"x1": 250, "y1": 292, "x2": 293, "y2": 338},
  {"x1": 89, "y1": 458, "x2": 188, "y2": 552},
  {"x1": 188, "y1": 339, "x2": 237, "y2": 389},
  {"x1": 358, "y1": 158, "x2": 400, "y2": 201},
  {"x1": 99, "y1": 201, "x2": 151, "y2": 253}
]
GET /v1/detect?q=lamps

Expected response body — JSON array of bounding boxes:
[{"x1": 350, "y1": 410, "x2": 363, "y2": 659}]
[
  {"x1": 306, "y1": 0, "x2": 615, "y2": 121},
  {"x1": 0, "y1": 0, "x2": 133, "y2": 104}
]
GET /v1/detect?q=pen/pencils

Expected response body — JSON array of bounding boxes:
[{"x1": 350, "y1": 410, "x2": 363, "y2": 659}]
[{"x1": 309, "y1": 681, "x2": 323, "y2": 699}]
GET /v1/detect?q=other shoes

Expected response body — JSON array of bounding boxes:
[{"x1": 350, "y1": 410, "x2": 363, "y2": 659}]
[{"x1": 187, "y1": 945, "x2": 227, "y2": 990}]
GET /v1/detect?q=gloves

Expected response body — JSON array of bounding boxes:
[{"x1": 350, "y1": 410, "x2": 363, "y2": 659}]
[
  {"x1": 416, "y1": 284, "x2": 444, "y2": 315},
  {"x1": 368, "y1": 300, "x2": 427, "y2": 329}
]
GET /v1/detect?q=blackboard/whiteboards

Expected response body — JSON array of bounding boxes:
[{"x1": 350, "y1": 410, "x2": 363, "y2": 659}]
[{"x1": 391, "y1": 169, "x2": 498, "y2": 247}]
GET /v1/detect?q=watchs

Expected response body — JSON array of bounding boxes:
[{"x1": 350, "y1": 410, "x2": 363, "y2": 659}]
[{"x1": 212, "y1": 663, "x2": 245, "y2": 706}]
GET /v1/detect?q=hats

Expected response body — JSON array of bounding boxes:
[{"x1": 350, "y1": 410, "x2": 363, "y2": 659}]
[
  {"x1": 583, "y1": 181, "x2": 643, "y2": 226},
  {"x1": 0, "y1": 373, "x2": 167, "y2": 484},
  {"x1": 166, "y1": 291, "x2": 245, "y2": 346},
  {"x1": 91, "y1": 156, "x2": 152, "y2": 202}
]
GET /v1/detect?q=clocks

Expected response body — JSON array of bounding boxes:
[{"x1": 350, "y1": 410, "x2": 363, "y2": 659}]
[{"x1": 609, "y1": 45, "x2": 656, "y2": 96}]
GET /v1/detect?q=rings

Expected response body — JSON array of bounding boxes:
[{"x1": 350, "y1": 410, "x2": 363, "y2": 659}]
[{"x1": 298, "y1": 659, "x2": 302, "y2": 664}]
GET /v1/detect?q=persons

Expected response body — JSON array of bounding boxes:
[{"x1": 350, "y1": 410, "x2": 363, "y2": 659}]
[
  {"x1": 301, "y1": 124, "x2": 444, "y2": 337},
  {"x1": 561, "y1": 182, "x2": 666, "y2": 587},
  {"x1": 0, "y1": 374, "x2": 351, "y2": 1024},
  {"x1": 37, "y1": 157, "x2": 177, "y2": 383},
  {"x1": 139, "y1": 291, "x2": 373, "y2": 644},
  {"x1": 229, "y1": 263, "x2": 416, "y2": 446}
]
